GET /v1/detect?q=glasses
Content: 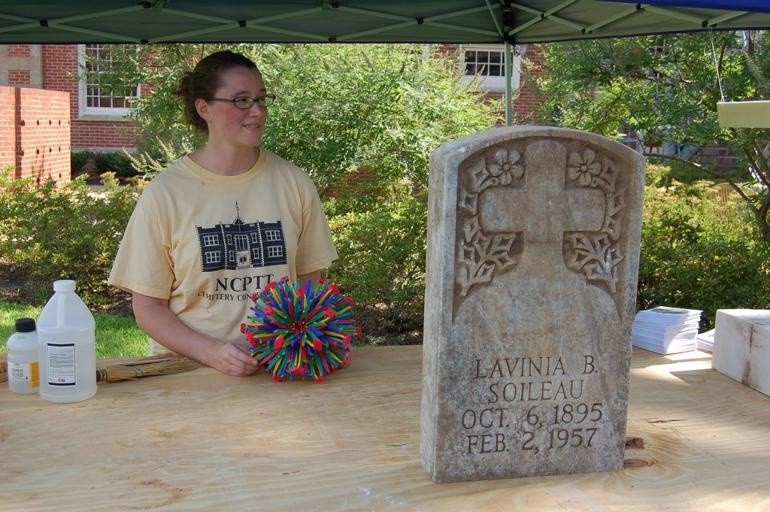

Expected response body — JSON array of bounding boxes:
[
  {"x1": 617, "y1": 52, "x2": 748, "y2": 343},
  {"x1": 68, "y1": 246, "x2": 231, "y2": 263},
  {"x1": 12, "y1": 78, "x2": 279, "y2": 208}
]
[{"x1": 203, "y1": 93, "x2": 276, "y2": 110}]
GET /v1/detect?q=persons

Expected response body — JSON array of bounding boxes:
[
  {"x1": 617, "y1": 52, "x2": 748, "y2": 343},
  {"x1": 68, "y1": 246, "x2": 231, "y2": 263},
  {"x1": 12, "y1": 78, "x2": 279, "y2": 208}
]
[{"x1": 106, "y1": 52, "x2": 342, "y2": 378}]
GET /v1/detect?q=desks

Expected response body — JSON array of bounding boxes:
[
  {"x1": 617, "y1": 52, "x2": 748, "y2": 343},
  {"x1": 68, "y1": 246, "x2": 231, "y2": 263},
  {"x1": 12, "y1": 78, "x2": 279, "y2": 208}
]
[{"x1": 0, "y1": 337, "x2": 770, "y2": 512}]
